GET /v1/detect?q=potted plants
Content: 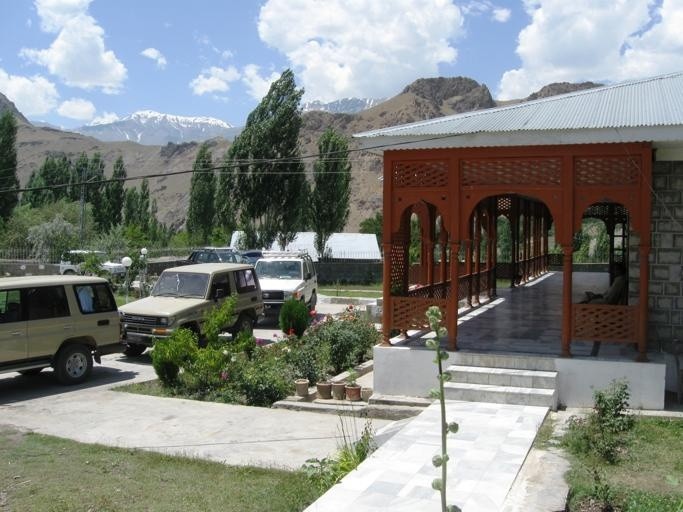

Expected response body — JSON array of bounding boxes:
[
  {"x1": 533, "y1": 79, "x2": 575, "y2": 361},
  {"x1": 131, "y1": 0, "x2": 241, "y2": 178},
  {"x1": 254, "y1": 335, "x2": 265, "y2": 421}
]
[
  {"x1": 343, "y1": 367, "x2": 361, "y2": 400},
  {"x1": 313, "y1": 365, "x2": 333, "y2": 399}
]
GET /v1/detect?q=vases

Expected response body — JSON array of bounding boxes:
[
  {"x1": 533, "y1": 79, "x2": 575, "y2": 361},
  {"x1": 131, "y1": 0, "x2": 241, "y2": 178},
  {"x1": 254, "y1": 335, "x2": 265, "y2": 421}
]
[
  {"x1": 330, "y1": 381, "x2": 346, "y2": 399},
  {"x1": 361, "y1": 388, "x2": 373, "y2": 403},
  {"x1": 295, "y1": 379, "x2": 311, "y2": 396}
]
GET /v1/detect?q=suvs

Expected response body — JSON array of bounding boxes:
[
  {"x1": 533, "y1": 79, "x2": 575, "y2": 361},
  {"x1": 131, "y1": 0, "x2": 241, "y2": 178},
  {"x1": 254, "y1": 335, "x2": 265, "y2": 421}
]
[
  {"x1": 245, "y1": 248, "x2": 318, "y2": 330},
  {"x1": 186, "y1": 249, "x2": 249, "y2": 264},
  {"x1": 117, "y1": 262, "x2": 264, "y2": 356},
  {"x1": 0, "y1": 274, "x2": 128, "y2": 388},
  {"x1": 59, "y1": 249, "x2": 124, "y2": 284}
]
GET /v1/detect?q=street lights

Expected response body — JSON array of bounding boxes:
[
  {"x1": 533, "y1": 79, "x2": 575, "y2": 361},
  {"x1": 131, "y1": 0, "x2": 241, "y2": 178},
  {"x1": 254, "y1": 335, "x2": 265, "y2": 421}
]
[{"x1": 121, "y1": 256, "x2": 132, "y2": 305}]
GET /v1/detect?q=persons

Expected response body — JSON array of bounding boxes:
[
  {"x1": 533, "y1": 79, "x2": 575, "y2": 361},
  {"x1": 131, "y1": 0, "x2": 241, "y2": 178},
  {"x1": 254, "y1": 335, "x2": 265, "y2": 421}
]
[{"x1": 576, "y1": 258, "x2": 623, "y2": 305}]
